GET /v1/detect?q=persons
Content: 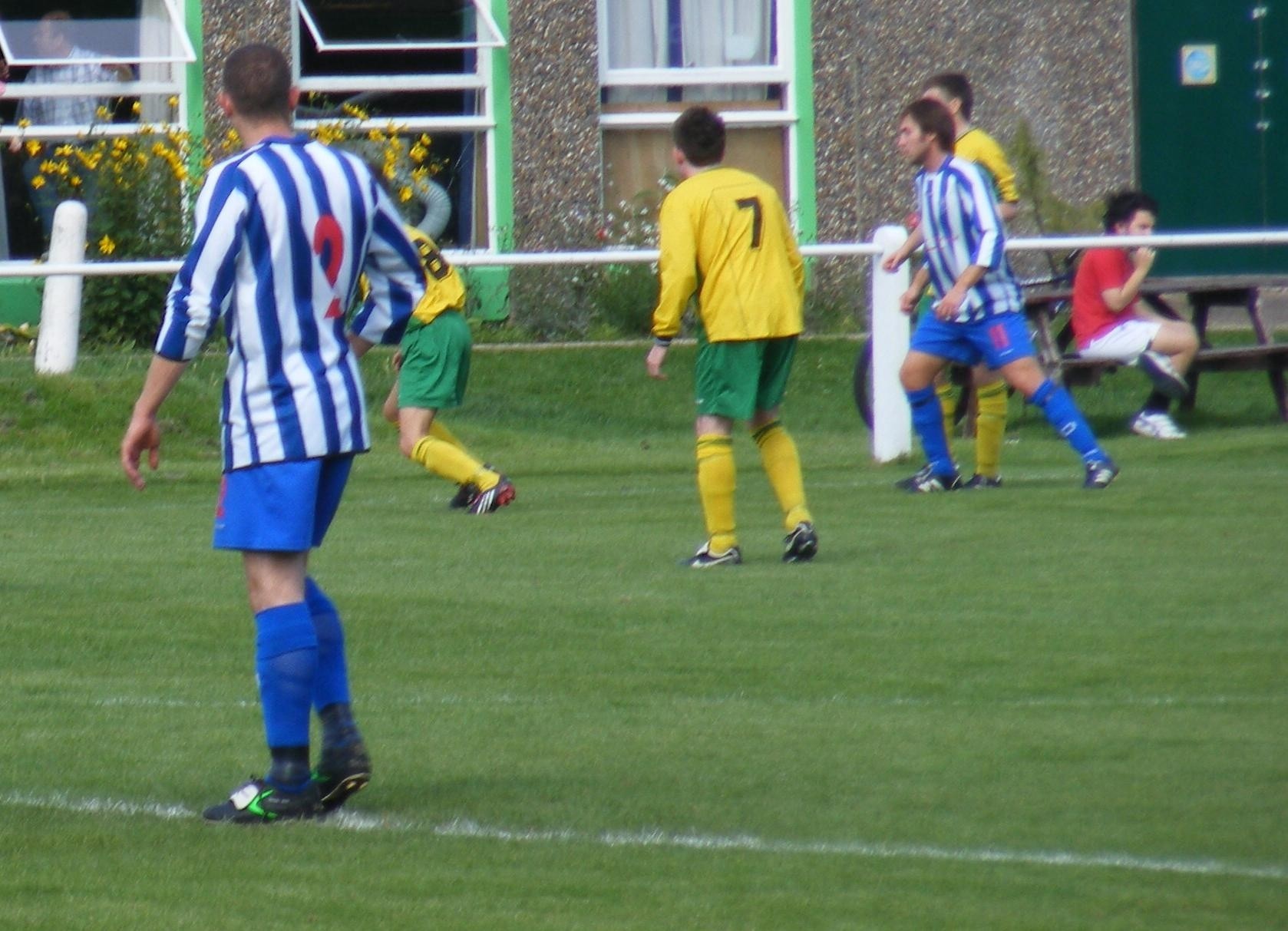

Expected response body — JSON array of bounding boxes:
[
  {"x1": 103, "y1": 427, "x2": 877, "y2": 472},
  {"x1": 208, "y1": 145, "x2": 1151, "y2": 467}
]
[
  {"x1": 358, "y1": 217, "x2": 516, "y2": 517},
  {"x1": 1072, "y1": 189, "x2": 1202, "y2": 441},
  {"x1": 123, "y1": 42, "x2": 430, "y2": 830},
  {"x1": 882, "y1": 72, "x2": 1118, "y2": 492},
  {"x1": 642, "y1": 105, "x2": 818, "y2": 568},
  {"x1": 0, "y1": 11, "x2": 135, "y2": 264}
]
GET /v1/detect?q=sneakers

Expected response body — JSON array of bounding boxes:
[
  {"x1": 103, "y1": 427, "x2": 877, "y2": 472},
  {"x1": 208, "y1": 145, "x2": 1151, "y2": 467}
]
[
  {"x1": 204, "y1": 775, "x2": 324, "y2": 826},
  {"x1": 451, "y1": 463, "x2": 506, "y2": 510},
  {"x1": 1084, "y1": 460, "x2": 1119, "y2": 490},
  {"x1": 963, "y1": 474, "x2": 1004, "y2": 491},
  {"x1": 1132, "y1": 411, "x2": 1187, "y2": 440},
  {"x1": 465, "y1": 475, "x2": 516, "y2": 514},
  {"x1": 1140, "y1": 351, "x2": 1187, "y2": 393},
  {"x1": 896, "y1": 465, "x2": 963, "y2": 495},
  {"x1": 311, "y1": 740, "x2": 374, "y2": 812},
  {"x1": 782, "y1": 521, "x2": 817, "y2": 563},
  {"x1": 676, "y1": 541, "x2": 743, "y2": 569}
]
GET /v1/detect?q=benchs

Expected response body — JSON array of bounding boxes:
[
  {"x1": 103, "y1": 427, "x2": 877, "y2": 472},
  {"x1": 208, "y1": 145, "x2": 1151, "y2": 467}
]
[{"x1": 955, "y1": 343, "x2": 1288, "y2": 387}]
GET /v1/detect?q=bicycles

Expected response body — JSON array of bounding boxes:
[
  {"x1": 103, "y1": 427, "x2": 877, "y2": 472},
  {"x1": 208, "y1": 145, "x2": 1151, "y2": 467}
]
[{"x1": 852, "y1": 248, "x2": 1080, "y2": 431}]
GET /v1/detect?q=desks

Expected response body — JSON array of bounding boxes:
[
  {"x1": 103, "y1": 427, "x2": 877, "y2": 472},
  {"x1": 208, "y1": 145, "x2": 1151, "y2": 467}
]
[{"x1": 953, "y1": 273, "x2": 1287, "y2": 427}]
